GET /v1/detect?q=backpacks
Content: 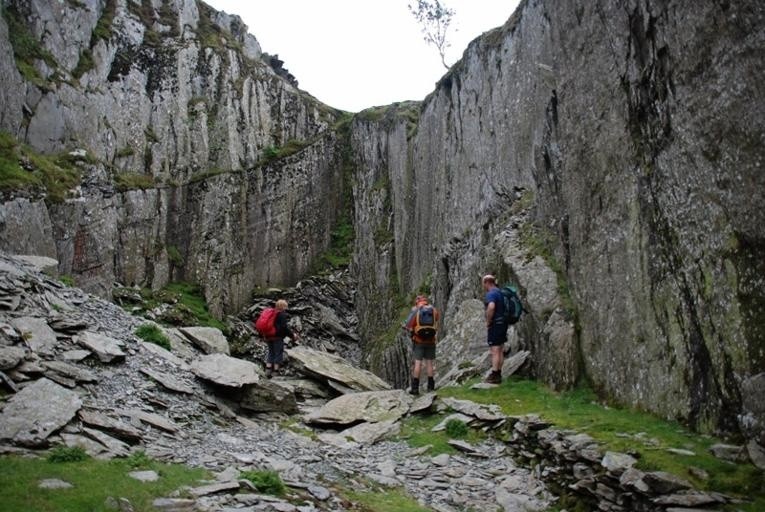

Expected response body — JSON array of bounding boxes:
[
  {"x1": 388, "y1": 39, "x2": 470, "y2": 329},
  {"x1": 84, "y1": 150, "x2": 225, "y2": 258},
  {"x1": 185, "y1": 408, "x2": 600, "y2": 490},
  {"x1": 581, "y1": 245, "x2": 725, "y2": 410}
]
[
  {"x1": 492, "y1": 285, "x2": 529, "y2": 325},
  {"x1": 256, "y1": 308, "x2": 282, "y2": 337},
  {"x1": 414, "y1": 304, "x2": 438, "y2": 340}
]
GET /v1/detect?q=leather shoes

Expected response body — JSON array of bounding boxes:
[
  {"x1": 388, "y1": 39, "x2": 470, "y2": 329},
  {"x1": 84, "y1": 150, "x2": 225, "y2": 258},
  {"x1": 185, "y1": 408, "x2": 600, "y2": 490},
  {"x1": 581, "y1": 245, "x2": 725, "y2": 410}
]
[
  {"x1": 409, "y1": 388, "x2": 419, "y2": 395},
  {"x1": 488, "y1": 376, "x2": 501, "y2": 383},
  {"x1": 488, "y1": 374, "x2": 495, "y2": 380}
]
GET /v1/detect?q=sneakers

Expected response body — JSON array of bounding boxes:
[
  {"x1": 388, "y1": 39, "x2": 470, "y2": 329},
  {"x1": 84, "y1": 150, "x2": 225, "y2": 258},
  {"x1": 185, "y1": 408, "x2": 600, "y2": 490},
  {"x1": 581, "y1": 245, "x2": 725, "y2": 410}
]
[
  {"x1": 265, "y1": 369, "x2": 272, "y2": 375},
  {"x1": 271, "y1": 372, "x2": 279, "y2": 376}
]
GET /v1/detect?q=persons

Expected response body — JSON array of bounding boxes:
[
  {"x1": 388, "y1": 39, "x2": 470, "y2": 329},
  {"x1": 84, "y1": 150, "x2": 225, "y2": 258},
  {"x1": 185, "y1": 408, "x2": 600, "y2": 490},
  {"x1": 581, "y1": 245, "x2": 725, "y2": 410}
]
[
  {"x1": 263, "y1": 298, "x2": 299, "y2": 377},
  {"x1": 480, "y1": 273, "x2": 508, "y2": 384},
  {"x1": 402, "y1": 292, "x2": 440, "y2": 390}
]
